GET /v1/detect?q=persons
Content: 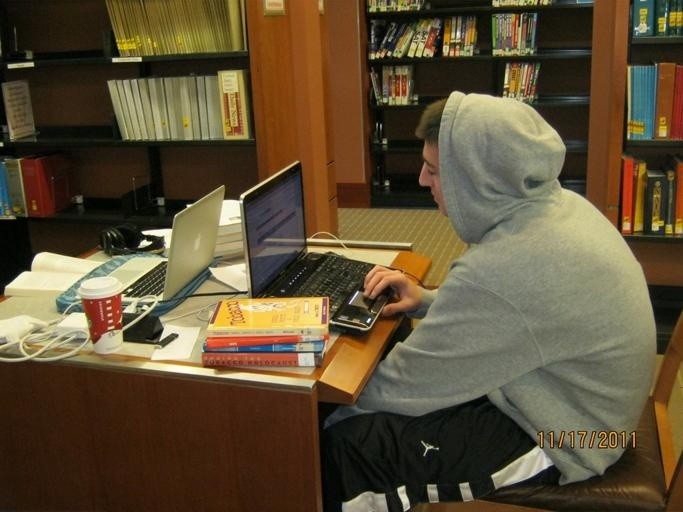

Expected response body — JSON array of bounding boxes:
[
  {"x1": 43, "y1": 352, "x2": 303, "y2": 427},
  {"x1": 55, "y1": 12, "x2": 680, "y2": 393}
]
[{"x1": 323, "y1": 92, "x2": 658, "y2": 511}]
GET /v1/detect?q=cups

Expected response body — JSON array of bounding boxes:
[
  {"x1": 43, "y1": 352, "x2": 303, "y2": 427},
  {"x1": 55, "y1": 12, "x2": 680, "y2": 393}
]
[{"x1": 76, "y1": 276, "x2": 124, "y2": 354}]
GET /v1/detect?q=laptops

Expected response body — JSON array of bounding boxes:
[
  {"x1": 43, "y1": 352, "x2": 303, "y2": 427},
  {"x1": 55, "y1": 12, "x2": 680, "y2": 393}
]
[
  {"x1": 239, "y1": 161, "x2": 404, "y2": 336},
  {"x1": 106, "y1": 185, "x2": 225, "y2": 305}
]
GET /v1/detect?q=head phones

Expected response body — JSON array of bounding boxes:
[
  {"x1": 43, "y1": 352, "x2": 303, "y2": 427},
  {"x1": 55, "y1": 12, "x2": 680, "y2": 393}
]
[{"x1": 101, "y1": 222, "x2": 165, "y2": 255}]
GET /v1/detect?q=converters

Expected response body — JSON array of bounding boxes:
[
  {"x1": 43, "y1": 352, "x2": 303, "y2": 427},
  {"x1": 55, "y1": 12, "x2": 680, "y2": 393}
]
[{"x1": 56, "y1": 312, "x2": 91, "y2": 339}]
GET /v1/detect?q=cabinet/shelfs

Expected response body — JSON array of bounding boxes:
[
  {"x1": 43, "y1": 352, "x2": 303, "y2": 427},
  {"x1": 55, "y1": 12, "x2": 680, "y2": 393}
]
[
  {"x1": 363, "y1": 1, "x2": 589, "y2": 206},
  {"x1": 608, "y1": 0, "x2": 683, "y2": 284},
  {"x1": 1, "y1": 0, "x2": 338, "y2": 292}
]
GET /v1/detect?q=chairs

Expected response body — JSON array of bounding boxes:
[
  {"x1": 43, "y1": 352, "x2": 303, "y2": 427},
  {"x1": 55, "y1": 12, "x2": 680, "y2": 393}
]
[{"x1": 388, "y1": 308, "x2": 683, "y2": 512}]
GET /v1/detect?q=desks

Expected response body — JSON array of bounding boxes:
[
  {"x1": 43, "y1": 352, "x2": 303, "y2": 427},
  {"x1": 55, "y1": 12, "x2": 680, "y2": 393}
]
[{"x1": 1, "y1": 232, "x2": 433, "y2": 512}]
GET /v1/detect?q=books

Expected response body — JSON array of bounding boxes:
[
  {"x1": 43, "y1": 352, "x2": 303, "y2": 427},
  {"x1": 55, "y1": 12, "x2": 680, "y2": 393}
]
[
  {"x1": 200, "y1": 296, "x2": 332, "y2": 369},
  {"x1": 2, "y1": 249, "x2": 106, "y2": 300},
  {"x1": 364, "y1": 0, "x2": 553, "y2": 110},
  {"x1": 101, "y1": 2, "x2": 251, "y2": 150},
  {"x1": 184, "y1": 198, "x2": 244, "y2": 265},
  {"x1": 0, "y1": 154, "x2": 78, "y2": 222},
  {"x1": 620, "y1": 0, "x2": 683, "y2": 239}
]
